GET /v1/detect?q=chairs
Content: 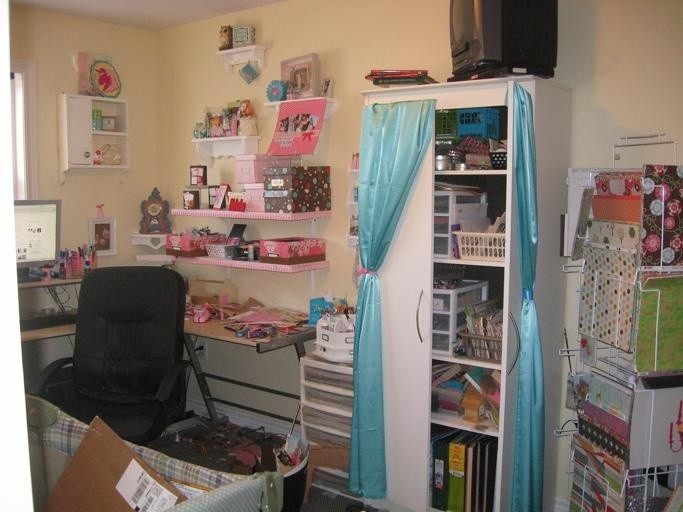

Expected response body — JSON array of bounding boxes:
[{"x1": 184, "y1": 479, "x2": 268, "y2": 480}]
[{"x1": 30, "y1": 265, "x2": 189, "y2": 448}]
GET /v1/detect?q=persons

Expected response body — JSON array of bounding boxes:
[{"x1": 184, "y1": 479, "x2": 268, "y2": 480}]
[{"x1": 292, "y1": 72, "x2": 306, "y2": 89}]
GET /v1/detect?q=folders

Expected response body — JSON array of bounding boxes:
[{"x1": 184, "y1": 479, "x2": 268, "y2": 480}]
[{"x1": 433, "y1": 430, "x2": 464, "y2": 511}]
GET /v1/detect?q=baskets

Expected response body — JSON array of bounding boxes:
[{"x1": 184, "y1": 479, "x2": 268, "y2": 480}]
[
  {"x1": 205, "y1": 243, "x2": 236, "y2": 258},
  {"x1": 451, "y1": 230, "x2": 506, "y2": 262},
  {"x1": 435, "y1": 110, "x2": 456, "y2": 145},
  {"x1": 458, "y1": 329, "x2": 501, "y2": 364},
  {"x1": 455, "y1": 109, "x2": 500, "y2": 143},
  {"x1": 488, "y1": 152, "x2": 506, "y2": 169}
]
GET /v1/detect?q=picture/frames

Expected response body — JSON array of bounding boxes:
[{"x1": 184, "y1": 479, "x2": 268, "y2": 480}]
[
  {"x1": 88, "y1": 217, "x2": 119, "y2": 258},
  {"x1": 281, "y1": 53, "x2": 319, "y2": 101}
]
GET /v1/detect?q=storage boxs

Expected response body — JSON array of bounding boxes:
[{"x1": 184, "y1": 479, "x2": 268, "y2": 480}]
[{"x1": 234, "y1": 152, "x2": 332, "y2": 216}]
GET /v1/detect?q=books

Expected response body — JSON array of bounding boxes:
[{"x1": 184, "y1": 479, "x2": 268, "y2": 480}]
[
  {"x1": 568, "y1": 163, "x2": 682, "y2": 510},
  {"x1": 430, "y1": 292, "x2": 503, "y2": 512},
  {"x1": 363, "y1": 68, "x2": 441, "y2": 88}
]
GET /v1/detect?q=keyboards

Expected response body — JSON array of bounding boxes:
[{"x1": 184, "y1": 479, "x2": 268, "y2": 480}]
[{"x1": 20, "y1": 313, "x2": 77, "y2": 333}]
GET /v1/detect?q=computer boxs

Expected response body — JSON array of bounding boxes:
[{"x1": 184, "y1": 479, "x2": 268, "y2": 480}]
[{"x1": 42, "y1": 365, "x2": 75, "y2": 405}]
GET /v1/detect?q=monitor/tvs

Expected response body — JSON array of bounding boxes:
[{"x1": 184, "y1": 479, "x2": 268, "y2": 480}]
[
  {"x1": 448, "y1": 0, "x2": 559, "y2": 78},
  {"x1": 13, "y1": 199, "x2": 62, "y2": 283}
]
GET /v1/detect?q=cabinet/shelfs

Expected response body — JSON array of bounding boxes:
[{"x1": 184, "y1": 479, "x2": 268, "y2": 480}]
[
  {"x1": 130, "y1": 45, "x2": 335, "y2": 270},
  {"x1": 361, "y1": 75, "x2": 573, "y2": 511},
  {"x1": 56, "y1": 92, "x2": 131, "y2": 173},
  {"x1": 299, "y1": 352, "x2": 356, "y2": 509}
]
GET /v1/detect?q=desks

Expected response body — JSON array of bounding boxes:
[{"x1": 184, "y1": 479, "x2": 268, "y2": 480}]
[{"x1": 21, "y1": 297, "x2": 316, "y2": 434}]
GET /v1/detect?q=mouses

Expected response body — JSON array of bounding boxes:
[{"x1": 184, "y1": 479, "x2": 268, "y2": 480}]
[{"x1": 43, "y1": 306, "x2": 56, "y2": 319}]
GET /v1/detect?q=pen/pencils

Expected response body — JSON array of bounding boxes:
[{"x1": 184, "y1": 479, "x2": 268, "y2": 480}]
[
  {"x1": 77, "y1": 242, "x2": 96, "y2": 258},
  {"x1": 224, "y1": 326, "x2": 236, "y2": 332}
]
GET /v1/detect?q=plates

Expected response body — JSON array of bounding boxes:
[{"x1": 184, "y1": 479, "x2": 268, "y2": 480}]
[{"x1": 88, "y1": 59, "x2": 121, "y2": 97}]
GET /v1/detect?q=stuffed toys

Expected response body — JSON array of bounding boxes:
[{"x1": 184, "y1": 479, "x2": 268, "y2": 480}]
[{"x1": 214, "y1": 24, "x2": 233, "y2": 51}]
